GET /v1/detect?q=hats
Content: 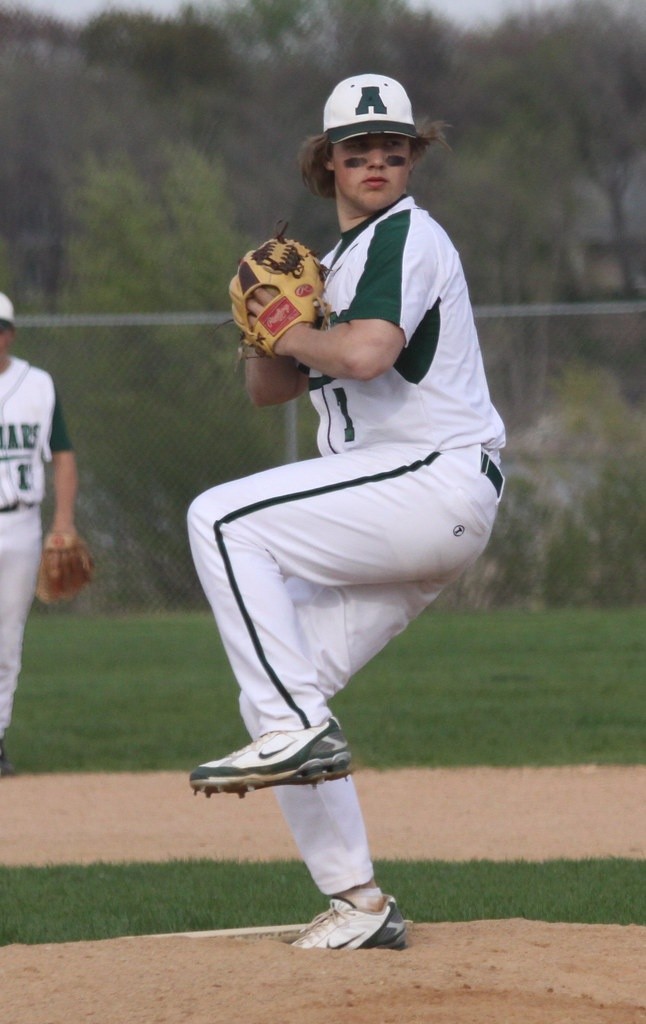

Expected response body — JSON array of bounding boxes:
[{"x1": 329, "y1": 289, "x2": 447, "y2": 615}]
[
  {"x1": 324, "y1": 74, "x2": 416, "y2": 143},
  {"x1": 0, "y1": 293, "x2": 14, "y2": 326}
]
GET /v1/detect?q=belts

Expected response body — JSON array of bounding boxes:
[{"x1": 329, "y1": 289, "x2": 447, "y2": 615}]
[
  {"x1": 0, "y1": 502, "x2": 35, "y2": 512},
  {"x1": 482, "y1": 452, "x2": 504, "y2": 499}
]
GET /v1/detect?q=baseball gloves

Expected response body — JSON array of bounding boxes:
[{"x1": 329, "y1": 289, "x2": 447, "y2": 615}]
[
  {"x1": 35, "y1": 529, "x2": 95, "y2": 605},
  {"x1": 207, "y1": 218, "x2": 334, "y2": 360}
]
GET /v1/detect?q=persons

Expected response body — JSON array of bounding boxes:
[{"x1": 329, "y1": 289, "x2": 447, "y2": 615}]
[
  {"x1": 188, "y1": 73, "x2": 509, "y2": 948},
  {"x1": 0, "y1": 294, "x2": 84, "y2": 773}
]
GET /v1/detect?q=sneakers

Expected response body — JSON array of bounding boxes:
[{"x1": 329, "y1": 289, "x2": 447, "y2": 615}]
[
  {"x1": 290, "y1": 895, "x2": 406, "y2": 950},
  {"x1": 190, "y1": 717, "x2": 352, "y2": 799}
]
